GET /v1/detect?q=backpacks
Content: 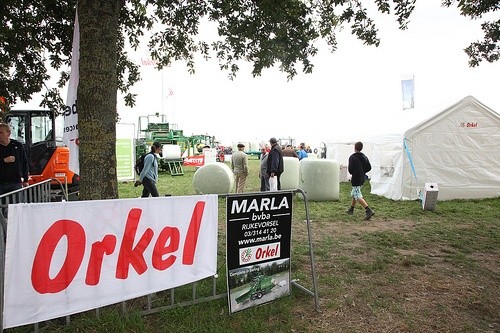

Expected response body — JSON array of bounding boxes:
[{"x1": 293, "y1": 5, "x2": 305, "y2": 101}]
[{"x1": 135, "y1": 151, "x2": 155, "y2": 176}]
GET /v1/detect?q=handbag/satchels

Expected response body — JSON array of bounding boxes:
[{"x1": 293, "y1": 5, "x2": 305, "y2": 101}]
[{"x1": 269, "y1": 175, "x2": 277, "y2": 191}]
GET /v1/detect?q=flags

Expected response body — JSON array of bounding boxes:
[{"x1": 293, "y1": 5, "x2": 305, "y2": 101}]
[
  {"x1": 63, "y1": 8, "x2": 79, "y2": 177},
  {"x1": 401, "y1": 79, "x2": 414, "y2": 110}
]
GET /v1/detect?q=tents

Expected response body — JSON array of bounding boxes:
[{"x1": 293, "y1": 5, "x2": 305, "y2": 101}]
[{"x1": 329, "y1": 94, "x2": 500, "y2": 202}]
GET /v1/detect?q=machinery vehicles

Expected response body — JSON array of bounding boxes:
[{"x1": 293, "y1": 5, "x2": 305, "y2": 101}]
[
  {"x1": 133, "y1": 112, "x2": 185, "y2": 176},
  {"x1": 185, "y1": 134, "x2": 225, "y2": 166},
  {"x1": 0, "y1": 110, "x2": 79, "y2": 193}
]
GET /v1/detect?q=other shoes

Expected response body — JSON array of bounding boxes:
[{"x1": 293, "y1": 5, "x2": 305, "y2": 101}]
[
  {"x1": 347, "y1": 207, "x2": 354, "y2": 214},
  {"x1": 364, "y1": 208, "x2": 375, "y2": 219}
]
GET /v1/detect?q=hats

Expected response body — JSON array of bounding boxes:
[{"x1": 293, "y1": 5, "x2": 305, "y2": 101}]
[
  {"x1": 269, "y1": 137, "x2": 276, "y2": 142},
  {"x1": 237, "y1": 143, "x2": 245, "y2": 147}
]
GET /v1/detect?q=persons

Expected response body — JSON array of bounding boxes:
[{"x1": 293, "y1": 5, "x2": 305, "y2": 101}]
[
  {"x1": 346, "y1": 141, "x2": 375, "y2": 220},
  {"x1": 266, "y1": 141, "x2": 326, "y2": 161},
  {"x1": 230, "y1": 144, "x2": 249, "y2": 193},
  {"x1": 0, "y1": 123, "x2": 28, "y2": 217},
  {"x1": 258, "y1": 146, "x2": 270, "y2": 191},
  {"x1": 266, "y1": 137, "x2": 284, "y2": 190},
  {"x1": 134, "y1": 141, "x2": 162, "y2": 198}
]
